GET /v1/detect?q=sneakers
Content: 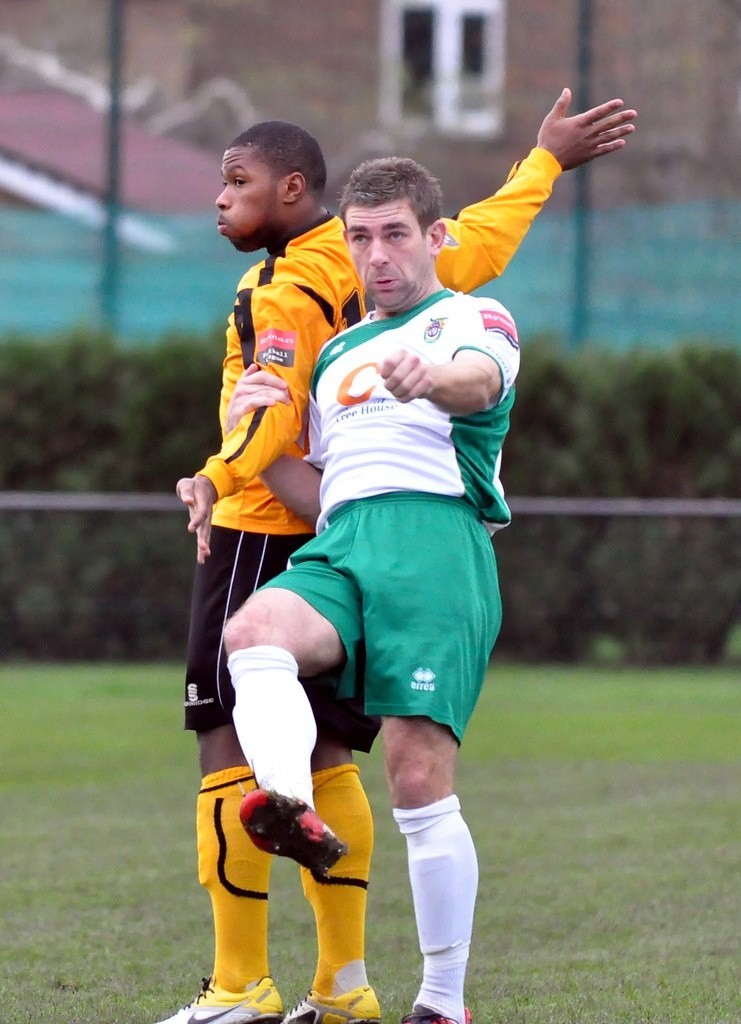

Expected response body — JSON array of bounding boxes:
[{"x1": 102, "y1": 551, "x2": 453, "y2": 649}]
[
  {"x1": 281, "y1": 986, "x2": 381, "y2": 1024},
  {"x1": 153, "y1": 972, "x2": 283, "y2": 1024},
  {"x1": 400, "y1": 1004, "x2": 473, "y2": 1024},
  {"x1": 238, "y1": 788, "x2": 342, "y2": 874}
]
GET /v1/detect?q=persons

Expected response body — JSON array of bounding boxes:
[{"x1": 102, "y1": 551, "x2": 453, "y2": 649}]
[
  {"x1": 154, "y1": 88, "x2": 639, "y2": 1024},
  {"x1": 223, "y1": 156, "x2": 520, "y2": 1024}
]
